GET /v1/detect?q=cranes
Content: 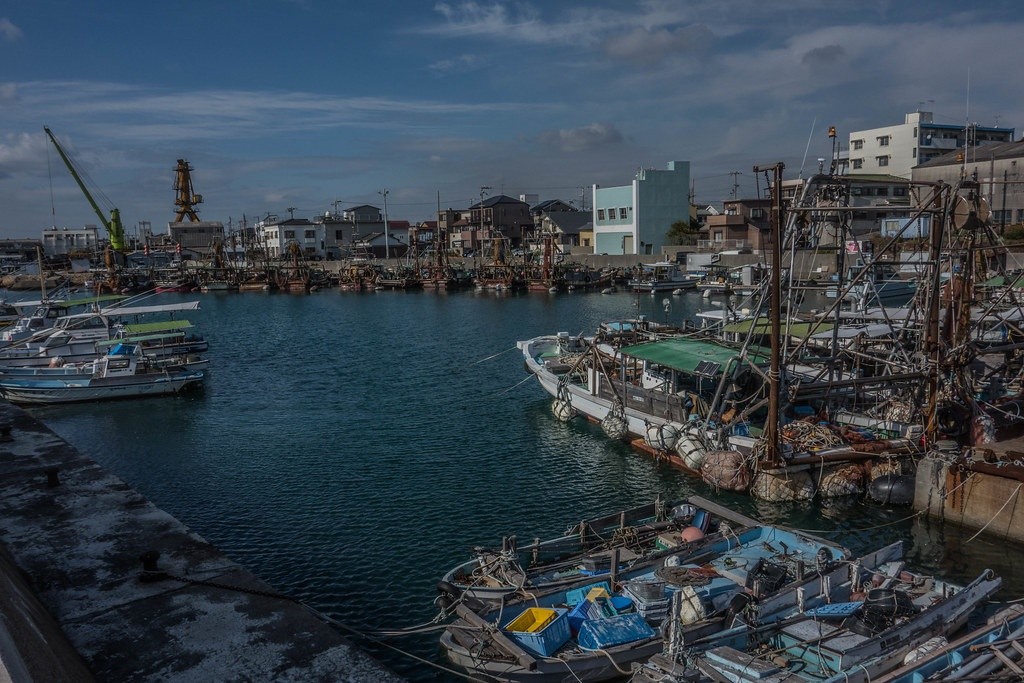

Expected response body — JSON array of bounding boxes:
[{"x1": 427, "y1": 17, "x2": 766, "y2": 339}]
[{"x1": 42, "y1": 123, "x2": 128, "y2": 251}]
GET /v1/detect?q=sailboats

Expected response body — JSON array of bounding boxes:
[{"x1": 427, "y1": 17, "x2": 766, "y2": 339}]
[
  {"x1": 470, "y1": 184, "x2": 518, "y2": 292},
  {"x1": 520, "y1": 114, "x2": 1024, "y2": 514},
  {"x1": 419, "y1": 189, "x2": 464, "y2": 291}
]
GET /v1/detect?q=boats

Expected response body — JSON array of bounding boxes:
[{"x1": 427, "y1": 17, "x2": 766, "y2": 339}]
[
  {"x1": 868, "y1": 601, "x2": 1023, "y2": 683},
  {"x1": 437, "y1": 494, "x2": 854, "y2": 683},
  {"x1": 627, "y1": 539, "x2": 1007, "y2": 682},
  {"x1": 0, "y1": 227, "x2": 421, "y2": 405},
  {"x1": 559, "y1": 262, "x2": 621, "y2": 293},
  {"x1": 626, "y1": 262, "x2": 706, "y2": 289},
  {"x1": 519, "y1": 227, "x2": 563, "y2": 293}
]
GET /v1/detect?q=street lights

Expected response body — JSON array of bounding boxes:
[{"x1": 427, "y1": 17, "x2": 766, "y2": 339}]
[{"x1": 376, "y1": 187, "x2": 391, "y2": 262}]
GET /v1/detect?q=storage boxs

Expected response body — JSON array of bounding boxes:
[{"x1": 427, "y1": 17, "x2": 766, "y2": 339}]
[
  {"x1": 499, "y1": 581, "x2": 654, "y2": 658},
  {"x1": 726, "y1": 421, "x2": 751, "y2": 436}
]
